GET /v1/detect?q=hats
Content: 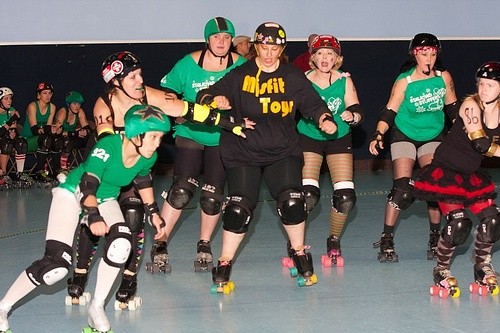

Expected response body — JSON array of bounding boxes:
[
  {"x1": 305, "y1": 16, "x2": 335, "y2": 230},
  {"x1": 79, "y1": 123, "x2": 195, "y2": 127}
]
[{"x1": 233, "y1": 35, "x2": 251, "y2": 47}]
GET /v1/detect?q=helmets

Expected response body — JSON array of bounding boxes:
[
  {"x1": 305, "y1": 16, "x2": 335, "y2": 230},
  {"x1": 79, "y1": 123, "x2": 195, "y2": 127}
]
[
  {"x1": 204, "y1": 17, "x2": 235, "y2": 43},
  {"x1": 37, "y1": 82, "x2": 54, "y2": 91},
  {"x1": 0, "y1": 87, "x2": 13, "y2": 100},
  {"x1": 312, "y1": 35, "x2": 341, "y2": 54},
  {"x1": 408, "y1": 33, "x2": 441, "y2": 55},
  {"x1": 253, "y1": 22, "x2": 286, "y2": 46},
  {"x1": 100, "y1": 51, "x2": 142, "y2": 84},
  {"x1": 124, "y1": 104, "x2": 171, "y2": 140},
  {"x1": 475, "y1": 61, "x2": 500, "y2": 83},
  {"x1": 65, "y1": 91, "x2": 84, "y2": 104}
]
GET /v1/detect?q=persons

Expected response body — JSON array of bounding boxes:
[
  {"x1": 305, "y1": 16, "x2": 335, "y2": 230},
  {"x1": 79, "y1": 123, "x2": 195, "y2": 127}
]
[
  {"x1": 367, "y1": 33, "x2": 457, "y2": 262},
  {"x1": 280, "y1": 34, "x2": 363, "y2": 269},
  {"x1": 0, "y1": 87, "x2": 36, "y2": 190},
  {"x1": 55, "y1": 90, "x2": 97, "y2": 174},
  {"x1": 144, "y1": 17, "x2": 248, "y2": 273},
  {"x1": 293, "y1": 34, "x2": 318, "y2": 73},
  {"x1": 196, "y1": 21, "x2": 338, "y2": 295},
  {"x1": 66, "y1": 50, "x2": 255, "y2": 313},
  {"x1": 21, "y1": 83, "x2": 67, "y2": 185},
  {"x1": 0, "y1": 105, "x2": 171, "y2": 333},
  {"x1": 232, "y1": 35, "x2": 252, "y2": 56},
  {"x1": 412, "y1": 62, "x2": 500, "y2": 297}
]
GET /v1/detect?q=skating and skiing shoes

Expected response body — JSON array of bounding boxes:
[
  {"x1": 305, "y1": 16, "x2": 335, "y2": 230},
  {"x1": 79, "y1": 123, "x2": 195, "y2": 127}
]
[
  {"x1": 373, "y1": 232, "x2": 399, "y2": 263},
  {"x1": 283, "y1": 240, "x2": 317, "y2": 287},
  {"x1": 321, "y1": 235, "x2": 345, "y2": 267},
  {"x1": 194, "y1": 241, "x2": 214, "y2": 271},
  {"x1": 37, "y1": 171, "x2": 55, "y2": 188},
  {"x1": 430, "y1": 265, "x2": 461, "y2": 298},
  {"x1": 114, "y1": 272, "x2": 142, "y2": 311},
  {"x1": 145, "y1": 241, "x2": 172, "y2": 274},
  {"x1": 13, "y1": 171, "x2": 35, "y2": 189},
  {"x1": 427, "y1": 231, "x2": 439, "y2": 260},
  {"x1": 0, "y1": 175, "x2": 14, "y2": 190},
  {"x1": 210, "y1": 259, "x2": 236, "y2": 293},
  {"x1": 469, "y1": 263, "x2": 500, "y2": 296},
  {"x1": 82, "y1": 300, "x2": 113, "y2": 333},
  {"x1": 0, "y1": 301, "x2": 12, "y2": 333},
  {"x1": 54, "y1": 170, "x2": 69, "y2": 183},
  {"x1": 65, "y1": 269, "x2": 91, "y2": 305}
]
